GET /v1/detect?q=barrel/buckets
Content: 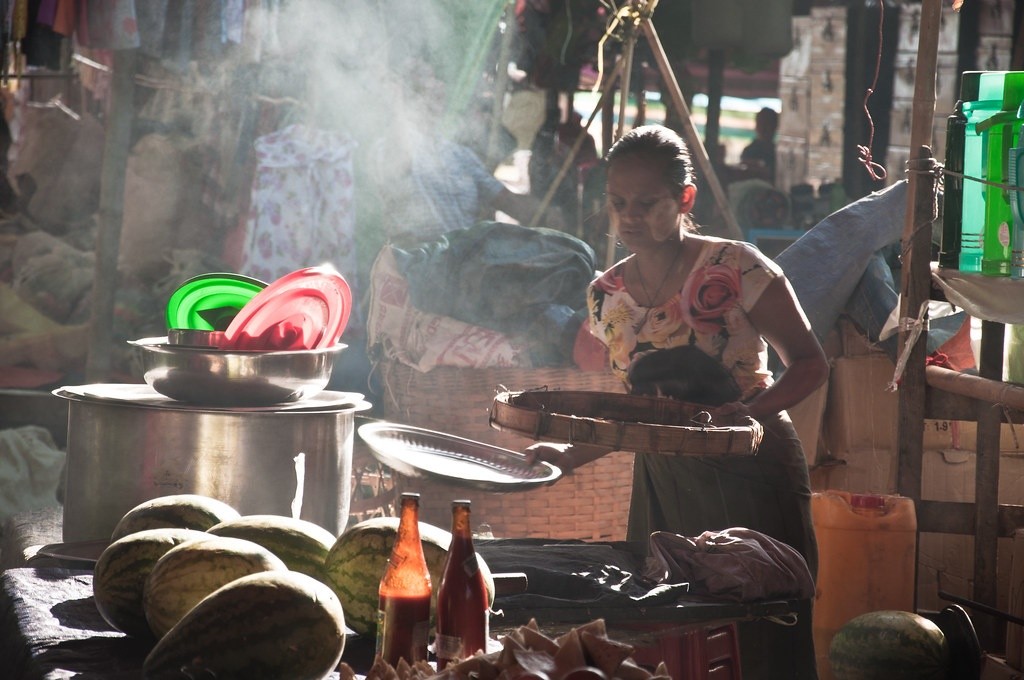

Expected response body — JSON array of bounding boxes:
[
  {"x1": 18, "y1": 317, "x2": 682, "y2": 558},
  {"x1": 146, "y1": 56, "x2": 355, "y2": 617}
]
[{"x1": 809, "y1": 490, "x2": 917, "y2": 680}]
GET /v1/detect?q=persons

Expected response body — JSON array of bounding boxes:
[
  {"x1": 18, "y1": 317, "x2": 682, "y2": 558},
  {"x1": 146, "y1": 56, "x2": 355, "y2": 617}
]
[
  {"x1": 239, "y1": 66, "x2": 910, "y2": 365},
  {"x1": 526, "y1": 124, "x2": 830, "y2": 680}
]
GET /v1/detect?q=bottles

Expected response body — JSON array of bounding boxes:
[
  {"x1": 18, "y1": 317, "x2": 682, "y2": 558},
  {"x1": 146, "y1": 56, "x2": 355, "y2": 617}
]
[
  {"x1": 434, "y1": 499, "x2": 490, "y2": 673},
  {"x1": 373, "y1": 492, "x2": 433, "y2": 668}
]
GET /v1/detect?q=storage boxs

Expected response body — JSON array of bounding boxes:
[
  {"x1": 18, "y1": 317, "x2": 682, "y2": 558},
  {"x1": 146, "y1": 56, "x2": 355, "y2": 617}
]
[{"x1": 770, "y1": 2, "x2": 1024, "y2": 680}]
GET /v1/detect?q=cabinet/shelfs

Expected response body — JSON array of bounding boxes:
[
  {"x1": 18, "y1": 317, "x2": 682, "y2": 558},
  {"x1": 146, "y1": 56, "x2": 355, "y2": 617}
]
[{"x1": 893, "y1": 1, "x2": 1024, "y2": 679}]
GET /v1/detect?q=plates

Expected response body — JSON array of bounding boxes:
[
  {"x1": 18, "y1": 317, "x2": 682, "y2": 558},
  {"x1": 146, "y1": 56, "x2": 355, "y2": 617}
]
[{"x1": 358, "y1": 421, "x2": 562, "y2": 493}]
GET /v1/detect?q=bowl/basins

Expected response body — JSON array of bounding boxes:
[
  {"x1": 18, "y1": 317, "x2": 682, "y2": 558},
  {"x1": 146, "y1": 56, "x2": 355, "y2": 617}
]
[{"x1": 123, "y1": 337, "x2": 349, "y2": 405}]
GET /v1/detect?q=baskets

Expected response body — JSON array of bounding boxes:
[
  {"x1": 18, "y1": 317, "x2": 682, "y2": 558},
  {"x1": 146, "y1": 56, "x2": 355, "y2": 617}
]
[{"x1": 488, "y1": 384, "x2": 763, "y2": 457}]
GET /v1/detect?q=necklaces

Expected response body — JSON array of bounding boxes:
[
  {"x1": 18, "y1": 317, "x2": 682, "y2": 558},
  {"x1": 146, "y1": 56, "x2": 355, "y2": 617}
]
[{"x1": 630, "y1": 250, "x2": 685, "y2": 335}]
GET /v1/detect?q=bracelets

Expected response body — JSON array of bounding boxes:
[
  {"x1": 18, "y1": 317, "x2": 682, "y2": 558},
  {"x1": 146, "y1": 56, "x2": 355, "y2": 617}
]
[{"x1": 564, "y1": 445, "x2": 581, "y2": 468}]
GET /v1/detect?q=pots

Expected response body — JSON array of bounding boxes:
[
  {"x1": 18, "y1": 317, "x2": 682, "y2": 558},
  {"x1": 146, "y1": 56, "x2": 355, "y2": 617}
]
[{"x1": 51, "y1": 383, "x2": 370, "y2": 540}]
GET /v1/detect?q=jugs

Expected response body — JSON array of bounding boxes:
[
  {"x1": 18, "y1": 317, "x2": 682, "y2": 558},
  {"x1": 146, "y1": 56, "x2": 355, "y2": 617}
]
[{"x1": 937, "y1": 70, "x2": 1024, "y2": 280}]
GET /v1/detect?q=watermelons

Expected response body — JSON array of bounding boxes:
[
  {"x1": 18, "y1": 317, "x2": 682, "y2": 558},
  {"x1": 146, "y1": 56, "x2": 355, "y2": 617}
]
[
  {"x1": 827, "y1": 610, "x2": 949, "y2": 680},
  {"x1": 92, "y1": 492, "x2": 495, "y2": 680}
]
